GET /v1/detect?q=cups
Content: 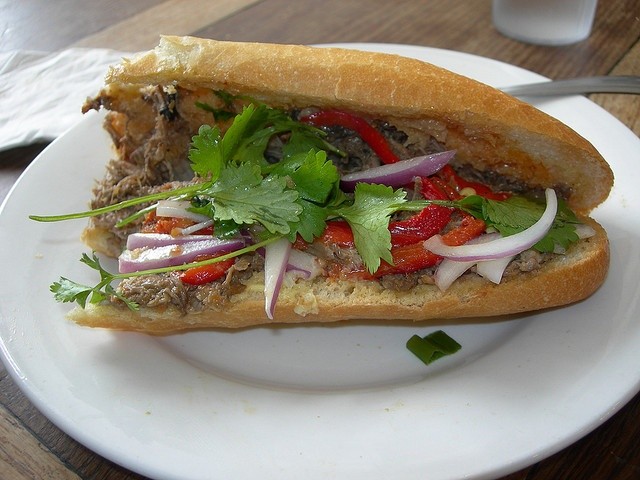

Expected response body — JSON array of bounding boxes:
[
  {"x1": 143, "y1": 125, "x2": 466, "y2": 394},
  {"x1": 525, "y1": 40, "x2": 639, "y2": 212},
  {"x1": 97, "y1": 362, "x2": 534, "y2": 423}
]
[{"x1": 491, "y1": 0, "x2": 597, "y2": 48}]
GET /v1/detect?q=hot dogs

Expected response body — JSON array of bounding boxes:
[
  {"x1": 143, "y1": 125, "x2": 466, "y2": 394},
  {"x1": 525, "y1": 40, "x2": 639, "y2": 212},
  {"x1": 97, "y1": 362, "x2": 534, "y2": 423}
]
[{"x1": 65, "y1": 35, "x2": 615, "y2": 334}]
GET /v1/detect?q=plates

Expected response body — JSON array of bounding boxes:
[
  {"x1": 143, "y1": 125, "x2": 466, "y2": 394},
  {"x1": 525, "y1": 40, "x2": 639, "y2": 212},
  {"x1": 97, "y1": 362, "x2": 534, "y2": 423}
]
[{"x1": 0, "y1": 41, "x2": 640, "y2": 480}]
